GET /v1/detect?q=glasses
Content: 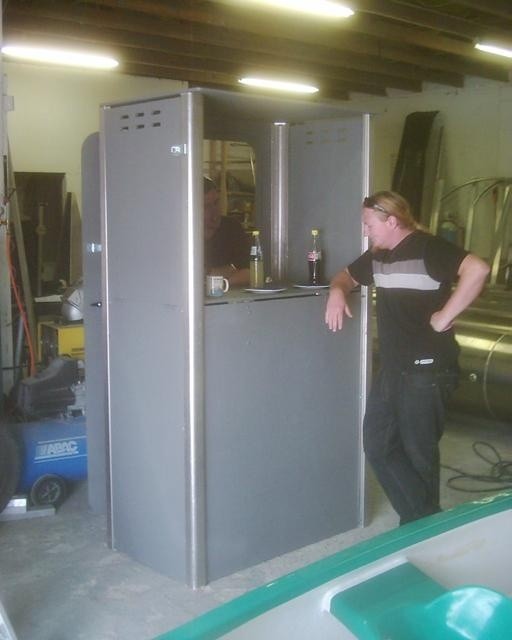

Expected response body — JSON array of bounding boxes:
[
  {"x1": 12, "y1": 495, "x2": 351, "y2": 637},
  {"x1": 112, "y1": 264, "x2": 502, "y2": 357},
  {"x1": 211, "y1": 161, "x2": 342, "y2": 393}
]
[{"x1": 364, "y1": 197, "x2": 386, "y2": 213}]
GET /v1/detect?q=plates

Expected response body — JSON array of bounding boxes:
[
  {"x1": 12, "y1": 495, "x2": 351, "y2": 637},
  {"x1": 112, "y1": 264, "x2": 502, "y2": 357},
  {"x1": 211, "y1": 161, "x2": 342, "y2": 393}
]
[
  {"x1": 294, "y1": 284, "x2": 332, "y2": 291},
  {"x1": 244, "y1": 287, "x2": 286, "y2": 296}
]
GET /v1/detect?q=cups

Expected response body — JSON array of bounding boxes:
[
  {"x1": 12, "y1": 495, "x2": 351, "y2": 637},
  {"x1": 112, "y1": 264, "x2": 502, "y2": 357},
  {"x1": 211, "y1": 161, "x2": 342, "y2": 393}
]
[{"x1": 207, "y1": 277, "x2": 228, "y2": 299}]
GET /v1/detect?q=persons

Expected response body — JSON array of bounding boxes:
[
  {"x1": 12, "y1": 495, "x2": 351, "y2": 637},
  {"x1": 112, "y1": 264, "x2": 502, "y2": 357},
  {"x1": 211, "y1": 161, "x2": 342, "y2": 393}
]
[
  {"x1": 322, "y1": 188, "x2": 493, "y2": 525},
  {"x1": 204, "y1": 176, "x2": 254, "y2": 286}
]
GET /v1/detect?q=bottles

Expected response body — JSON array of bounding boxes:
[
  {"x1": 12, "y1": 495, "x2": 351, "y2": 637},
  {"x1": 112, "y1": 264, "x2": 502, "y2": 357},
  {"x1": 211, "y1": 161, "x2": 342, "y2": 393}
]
[
  {"x1": 249, "y1": 231, "x2": 264, "y2": 289},
  {"x1": 308, "y1": 229, "x2": 321, "y2": 285}
]
